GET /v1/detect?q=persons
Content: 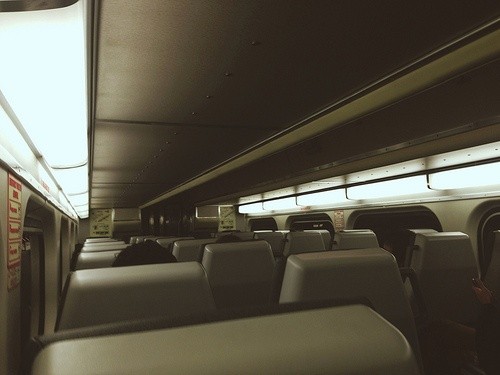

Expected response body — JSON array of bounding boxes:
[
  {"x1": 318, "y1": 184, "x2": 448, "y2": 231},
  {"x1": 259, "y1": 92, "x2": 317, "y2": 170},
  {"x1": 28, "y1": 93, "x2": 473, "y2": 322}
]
[
  {"x1": 471, "y1": 275, "x2": 500, "y2": 375},
  {"x1": 111, "y1": 240, "x2": 179, "y2": 266}
]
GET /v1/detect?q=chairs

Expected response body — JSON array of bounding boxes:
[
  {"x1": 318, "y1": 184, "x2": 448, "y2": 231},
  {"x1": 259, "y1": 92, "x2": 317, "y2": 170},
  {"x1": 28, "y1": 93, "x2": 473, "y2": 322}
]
[{"x1": 20, "y1": 227, "x2": 500, "y2": 375}]
